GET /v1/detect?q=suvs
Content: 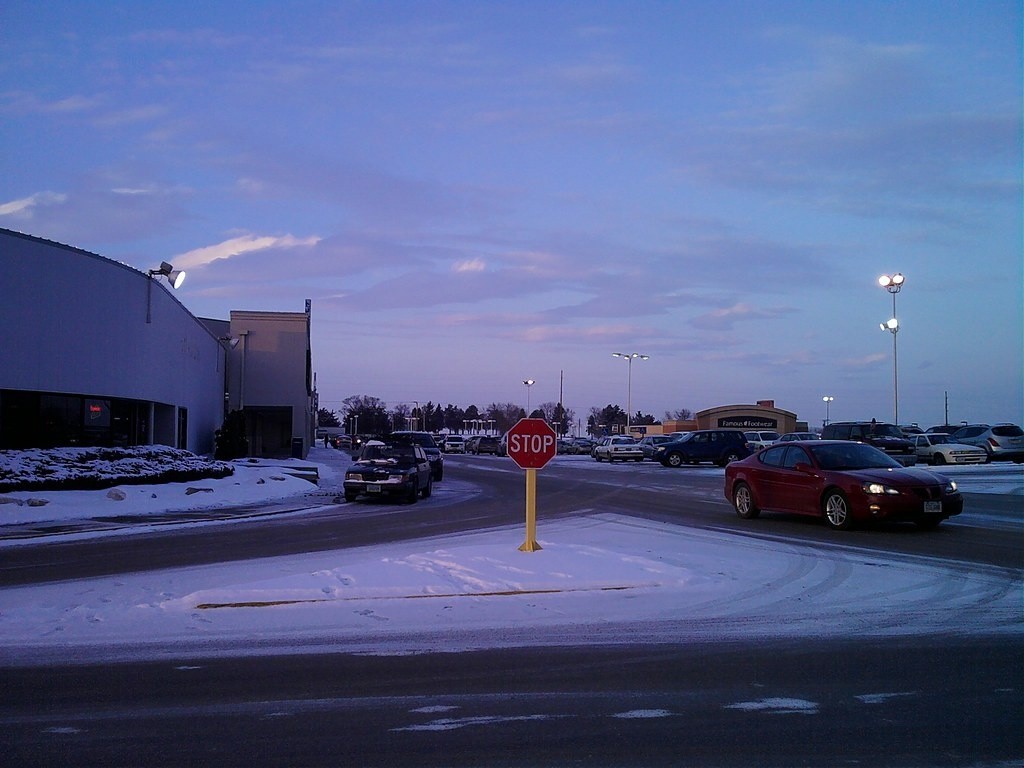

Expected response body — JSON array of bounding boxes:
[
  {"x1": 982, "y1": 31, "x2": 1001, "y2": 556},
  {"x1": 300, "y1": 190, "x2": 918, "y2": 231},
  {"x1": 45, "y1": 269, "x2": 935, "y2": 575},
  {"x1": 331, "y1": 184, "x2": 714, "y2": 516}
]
[
  {"x1": 444, "y1": 434, "x2": 465, "y2": 454},
  {"x1": 819, "y1": 420, "x2": 918, "y2": 468},
  {"x1": 391, "y1": 431, "x2": 444, "y2": 482},
  {"x1": 651, "y1": 430, "x2": 750, "y2": 468},
  {"x1": 742, "y1": 431, "x2": 781, "y2": 455},
  {"x1": 670, "y1": 432, "x2": 698, "y2": 442}
]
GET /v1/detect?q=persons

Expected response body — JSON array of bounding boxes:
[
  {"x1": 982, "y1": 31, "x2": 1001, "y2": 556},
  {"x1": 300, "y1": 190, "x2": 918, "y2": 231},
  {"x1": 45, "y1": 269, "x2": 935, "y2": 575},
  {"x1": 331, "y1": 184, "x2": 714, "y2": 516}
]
[{"x1": 324, "y1": 432, "x2": 328, "y2": 448}]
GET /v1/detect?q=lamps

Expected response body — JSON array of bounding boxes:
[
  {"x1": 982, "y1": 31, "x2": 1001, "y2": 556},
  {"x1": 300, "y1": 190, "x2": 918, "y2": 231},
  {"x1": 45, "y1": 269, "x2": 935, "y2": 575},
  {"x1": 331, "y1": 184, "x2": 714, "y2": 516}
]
[
  {"x1": 217, "y1": 333, "x2": 240, "y2": 349},
  {"x1": 148, "y1": 261, "x2": 185, "y2": 290}
]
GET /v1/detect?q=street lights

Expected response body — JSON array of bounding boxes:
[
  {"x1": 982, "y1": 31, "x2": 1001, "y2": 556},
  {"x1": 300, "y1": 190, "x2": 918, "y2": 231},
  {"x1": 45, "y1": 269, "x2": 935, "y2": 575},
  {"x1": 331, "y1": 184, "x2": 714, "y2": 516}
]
[
  {"x1": 613, "y1": 352, "x2": 650, "y2": 433},
  {"x1": 350, "y1": 417, "x2": 353, "y2": 436},
  {"x1": 823, "y1": 396, "x2": 833, "y2": 425},
  {"x1": 522, "y1": 379, "x2": 535, "y2": 417},
  {"x1": 354, "y1": 415, "x2": 359, "y2": 436},
  {"x1": 877, "y1": 273, "x2": 901, "y2": 425}
]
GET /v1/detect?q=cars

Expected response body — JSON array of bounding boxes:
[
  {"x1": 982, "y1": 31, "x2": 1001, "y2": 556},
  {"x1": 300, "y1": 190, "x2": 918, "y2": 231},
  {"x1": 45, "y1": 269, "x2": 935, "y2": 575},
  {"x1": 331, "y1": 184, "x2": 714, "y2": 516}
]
[
  {"x1": 776, "y1": 432, "x2": 819, "y2": 442},
  {"x1": 924, "y1": 425, "x2": 962, "y2": 435},
  {"x1": 901, "y1": 426, "x2": 924, "y2": 437},
  {"x1": 343, "y1": 442, "x2": 433, "y2": 504},
  {"x1": 331, "y1": 434, "x2": 389, "y2": 461},
  {"x1": 722, "y1": 440, "x2": 964, "y2": 530},
  {"x1": 906, "y1": 433, "x2": 988, "y2": 465},
  {"x1": 638, "y1": 435, "x2": 674, "y2": 458},
  {"x1": 952, "y1": 422, "x2": 1024, "y2": 464},
  {"x1": 595, "y1": 436, "x2": 644, "y2": 463},
  {"x1": 466, "y1": 434, "x2": 509, "y2": 458},
  {"x1": 556, "y1": 439, "x2": 599, "y2": 455}
]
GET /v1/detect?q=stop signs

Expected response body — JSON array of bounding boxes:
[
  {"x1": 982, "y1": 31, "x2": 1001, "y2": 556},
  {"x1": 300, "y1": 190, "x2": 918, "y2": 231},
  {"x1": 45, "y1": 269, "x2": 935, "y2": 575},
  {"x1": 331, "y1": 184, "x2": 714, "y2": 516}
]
[{"x1": 507, "y1": 418, "x2": 558, "y2": 469}]
